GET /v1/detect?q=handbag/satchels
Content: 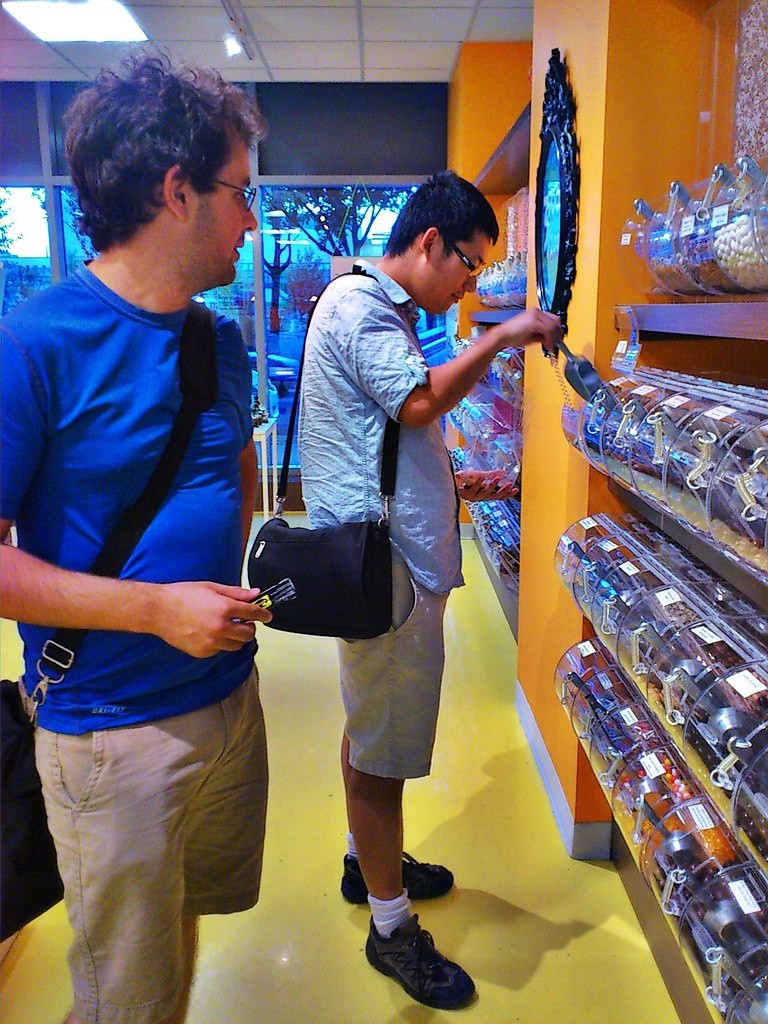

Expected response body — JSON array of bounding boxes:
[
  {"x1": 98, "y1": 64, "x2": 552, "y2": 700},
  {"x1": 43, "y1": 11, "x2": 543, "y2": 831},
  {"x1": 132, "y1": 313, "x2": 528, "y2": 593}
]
[
  {"x1": 250, "y1": 513, "x2": 395, "y2": 640},
  {"x1": 1, "y1": 681, "x2": 62, "y2": 945}
]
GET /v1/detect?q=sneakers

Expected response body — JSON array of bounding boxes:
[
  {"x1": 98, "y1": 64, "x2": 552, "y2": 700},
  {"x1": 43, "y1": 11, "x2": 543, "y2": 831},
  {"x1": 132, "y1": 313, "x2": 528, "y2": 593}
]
[
  {"x1": 342, "y1": 851, "x2": 455, "y2": 905},
  {"x1": 365, "y1": 914, "x2": 477, "y2": 1010}
]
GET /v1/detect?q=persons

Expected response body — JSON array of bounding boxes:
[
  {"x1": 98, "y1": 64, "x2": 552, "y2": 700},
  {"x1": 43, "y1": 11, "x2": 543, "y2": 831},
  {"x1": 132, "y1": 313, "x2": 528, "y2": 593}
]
[
  {"x1": 296, "y1": 170, "x2": 563, "y2": 1011},
  {"x1": 0, "y1": 60, "x2": 269, "y2": 1024}
]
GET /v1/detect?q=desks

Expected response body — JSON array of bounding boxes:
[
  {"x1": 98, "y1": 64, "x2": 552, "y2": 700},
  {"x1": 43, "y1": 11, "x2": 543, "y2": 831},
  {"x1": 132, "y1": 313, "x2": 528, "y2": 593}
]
[{"x1": 253, "y1": 418, "x2": 279, "y2": 525}]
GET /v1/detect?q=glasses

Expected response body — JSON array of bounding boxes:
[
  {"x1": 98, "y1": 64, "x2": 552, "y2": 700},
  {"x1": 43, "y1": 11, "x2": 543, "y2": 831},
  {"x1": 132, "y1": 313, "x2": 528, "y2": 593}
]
[
  {"x1": 425, "y1": 222, "x2": 485, "y2": 279},
  {"x1": 183, "y1": 165, "x2": 256, "y2": 209}
]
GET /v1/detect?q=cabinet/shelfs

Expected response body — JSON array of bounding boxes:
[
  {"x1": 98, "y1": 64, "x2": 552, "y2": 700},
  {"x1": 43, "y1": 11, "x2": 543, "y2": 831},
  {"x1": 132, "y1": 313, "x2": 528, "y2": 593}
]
[
  {"x1": 449, "y1": 101, "x2": 530, "y2": 641},
  {"x1": 552, "y1": 294, "x2": 768, "y2": 1024}
]
